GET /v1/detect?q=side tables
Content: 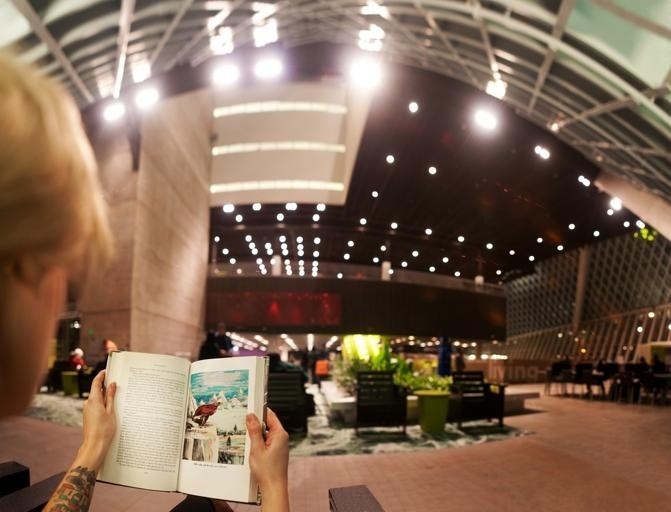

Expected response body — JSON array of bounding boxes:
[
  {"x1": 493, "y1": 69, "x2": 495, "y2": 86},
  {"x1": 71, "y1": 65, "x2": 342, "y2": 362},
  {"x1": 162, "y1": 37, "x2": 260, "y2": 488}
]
[{"x1": 413, "y1": 388, "x2": 451, "y2": 436}]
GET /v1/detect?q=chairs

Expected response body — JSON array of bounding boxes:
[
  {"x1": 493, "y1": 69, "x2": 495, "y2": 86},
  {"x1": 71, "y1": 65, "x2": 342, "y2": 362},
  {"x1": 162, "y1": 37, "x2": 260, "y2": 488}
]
[
  {"x1": 543, "y1": 359, "x2": 671, "y2": 407},
  {"x1": 447, "y1": 370, "x2": 509, "y2": 432},
  {"x1": 353, "y1": 369, "x2": 414, "y2": 439},
  {"x1": 266, "y1": 371, "x2": 310, "y2": 434},
  {"x1": 46, "y1": 361, "x2": 106, "y2": 397}
]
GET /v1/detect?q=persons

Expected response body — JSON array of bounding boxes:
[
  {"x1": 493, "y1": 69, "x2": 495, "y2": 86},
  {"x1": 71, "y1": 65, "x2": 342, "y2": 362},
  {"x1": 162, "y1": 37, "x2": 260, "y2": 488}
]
[
  {"x1": 651, "y1": 354, "x2": 666, "y2": 375},
  {"x1": 1, "y1": 47, "x2": 290, "y2": 512},
  {"x1": 637, "y1": 356, "x2": 649, "y2": 372},
  {"x1": 91, "y1": 353, "x2": 108, "y2": 377},
  {"x1": 197, "y1": 329, "x2": 228, "y2": 358},
  {"x1": 265, "y1": 342, "x2": 338, "y2": 389},
  {"x1": 214, "y1": 320, "x2": 233, "y2": 354},
  {"x1": 68, "y1": 347, "x2": 88, "y2": 372}
]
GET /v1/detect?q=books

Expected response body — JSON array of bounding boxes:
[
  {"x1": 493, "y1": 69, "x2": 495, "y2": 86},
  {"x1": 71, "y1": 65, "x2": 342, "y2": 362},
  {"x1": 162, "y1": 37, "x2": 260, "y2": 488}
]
[{"x1": 90, "y1": 352, "x2": 270, "y2": 507}]
